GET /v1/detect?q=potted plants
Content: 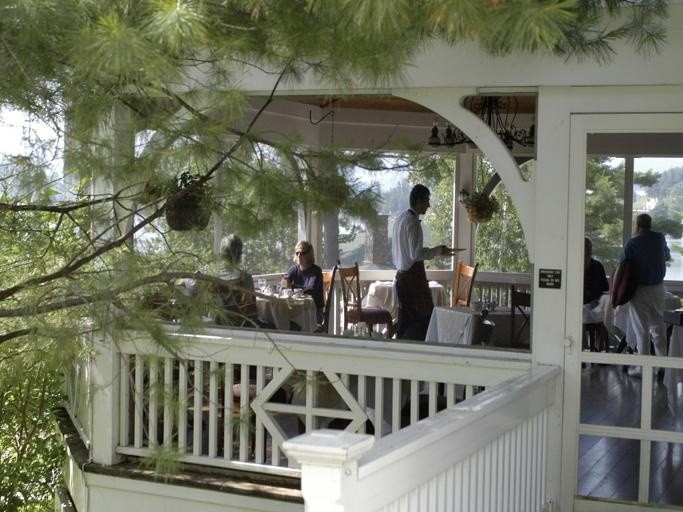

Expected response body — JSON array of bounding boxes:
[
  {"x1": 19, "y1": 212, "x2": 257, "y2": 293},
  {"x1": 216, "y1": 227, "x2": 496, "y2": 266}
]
[{"x1": 459, "y1": 191, "x2": 505, "y2": 224}]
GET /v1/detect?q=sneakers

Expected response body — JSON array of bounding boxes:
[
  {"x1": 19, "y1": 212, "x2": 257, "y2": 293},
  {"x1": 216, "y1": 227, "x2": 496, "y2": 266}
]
[{"x1": 628, "y1": 365, "x2": 644, "y2": 379}]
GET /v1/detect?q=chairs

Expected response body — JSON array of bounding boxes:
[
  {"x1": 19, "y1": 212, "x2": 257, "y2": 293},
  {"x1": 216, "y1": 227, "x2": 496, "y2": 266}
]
[
  {"x1": 321, "y1": 257, "x2": 393, "y2": 337},
  {"x1": 454, "y1": 259, "x2": 480, "y2": 307}
]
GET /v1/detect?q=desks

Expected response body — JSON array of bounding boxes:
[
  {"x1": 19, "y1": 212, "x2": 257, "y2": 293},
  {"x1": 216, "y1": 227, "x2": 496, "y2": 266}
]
[{"x1": 600, "y1": 290, "x2": 680, "y2": 353}]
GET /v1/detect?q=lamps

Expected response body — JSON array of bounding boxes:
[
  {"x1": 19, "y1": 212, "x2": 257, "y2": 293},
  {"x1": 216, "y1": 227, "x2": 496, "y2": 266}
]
[
  {"x1": 459, "y1": 187, "x2": 468, "y2": 205},
  {"x1": 428, "y1": 94, "x2": 535, "y2": 152}
]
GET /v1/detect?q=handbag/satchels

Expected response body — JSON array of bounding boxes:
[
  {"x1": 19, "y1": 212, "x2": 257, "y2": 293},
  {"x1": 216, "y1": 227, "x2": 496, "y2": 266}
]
[{"x1": 609, "y1": 262, "x2": 639, "y2": 306}]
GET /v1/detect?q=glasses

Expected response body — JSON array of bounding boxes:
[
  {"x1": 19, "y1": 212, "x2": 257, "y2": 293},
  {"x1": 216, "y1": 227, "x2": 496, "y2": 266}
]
[{"x1": 295, "y1": 251, "x2": 310, "y2": 256}]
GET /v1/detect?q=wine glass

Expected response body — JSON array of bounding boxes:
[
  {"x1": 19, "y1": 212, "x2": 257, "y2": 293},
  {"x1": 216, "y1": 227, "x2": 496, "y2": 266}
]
[{"x1": 258, "y1": 278, "x2": 266, "y2": 293}]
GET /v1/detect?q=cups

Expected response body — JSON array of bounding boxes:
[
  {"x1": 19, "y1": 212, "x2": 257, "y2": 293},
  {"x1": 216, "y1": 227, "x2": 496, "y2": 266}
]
[
  {"x1": 294, "y1": 288, "x2": 303, "y2": 297},
  {"x1": 470, "y1": 301, "x2": 482, "y2": 311},
  {"x1": 283, "y1": 288, "x2": 292, "y2": 297}
]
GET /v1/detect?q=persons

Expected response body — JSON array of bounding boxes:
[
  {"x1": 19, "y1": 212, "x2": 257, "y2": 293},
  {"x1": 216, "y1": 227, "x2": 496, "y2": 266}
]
[
  {"x1": 189, "y1": 234, "x2": 274, "y2": 330},
  {"x1": 391, "y1": 184, "x2": 456, "y2": 341},
  {"x1": 582, "y1": 237, "x2": 611, "y2": 369},
  {"x1": 616, "y1": 214, "x2": 670, "y2": 380},
  {"x1": 277, "y1": 240, "x2": 324, "y2": 324}
]
